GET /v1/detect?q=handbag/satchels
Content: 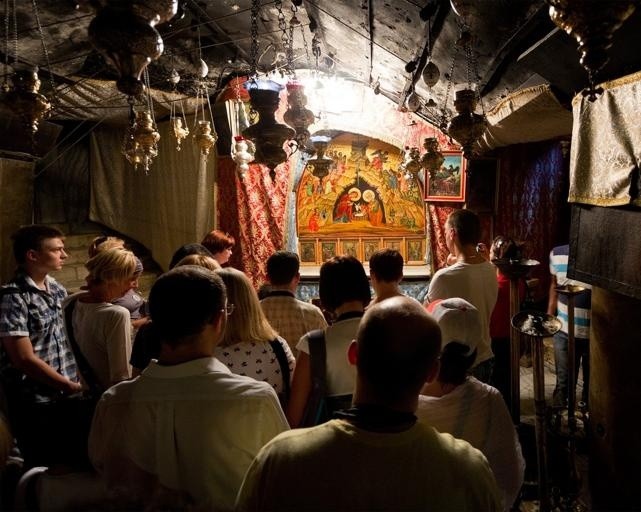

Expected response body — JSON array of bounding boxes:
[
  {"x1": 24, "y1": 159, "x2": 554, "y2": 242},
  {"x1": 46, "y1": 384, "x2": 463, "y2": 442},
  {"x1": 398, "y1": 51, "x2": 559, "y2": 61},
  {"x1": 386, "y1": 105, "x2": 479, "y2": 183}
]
[{"x1": 0, "y1": 367, "x2": 65, "y2": 428}]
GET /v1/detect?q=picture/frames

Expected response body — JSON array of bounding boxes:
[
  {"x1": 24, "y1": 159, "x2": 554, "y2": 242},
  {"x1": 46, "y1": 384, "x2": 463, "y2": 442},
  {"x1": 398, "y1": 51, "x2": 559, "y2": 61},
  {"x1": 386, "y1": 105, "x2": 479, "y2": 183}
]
[{"x1": 423, "y1": 149, "x2": 467, "y2": 203}]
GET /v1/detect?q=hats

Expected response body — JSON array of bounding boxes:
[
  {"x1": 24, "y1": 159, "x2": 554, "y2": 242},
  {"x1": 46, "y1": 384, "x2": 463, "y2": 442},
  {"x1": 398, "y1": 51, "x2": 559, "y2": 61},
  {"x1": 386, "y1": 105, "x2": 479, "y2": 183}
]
[{"x1": 423, "y1": 296, "x2": 483, "y2": 358}]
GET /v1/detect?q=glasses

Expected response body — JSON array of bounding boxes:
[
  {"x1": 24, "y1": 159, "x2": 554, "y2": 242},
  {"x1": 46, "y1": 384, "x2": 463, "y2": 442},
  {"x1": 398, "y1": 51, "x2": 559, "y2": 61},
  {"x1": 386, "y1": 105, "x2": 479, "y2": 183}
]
[{"x1": 221, "y1": 304, "x2": 235, "y2": 315}]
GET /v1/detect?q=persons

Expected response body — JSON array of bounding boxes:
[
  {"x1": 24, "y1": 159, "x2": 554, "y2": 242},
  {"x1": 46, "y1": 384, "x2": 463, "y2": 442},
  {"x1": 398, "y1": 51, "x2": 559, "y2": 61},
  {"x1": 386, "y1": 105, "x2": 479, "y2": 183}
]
[
  {"x1": 300, "y1": 147, "x2": 416, "y2": 232},
  {"x1": 543, "y1": 243, "x2": 593, "y2": 412},
  {"x1": 1, "y1": 208, "x2": 526, "y2": 511}
]
